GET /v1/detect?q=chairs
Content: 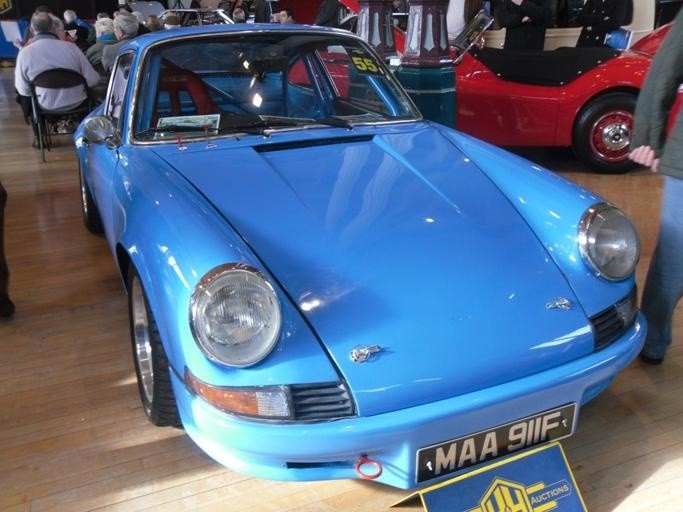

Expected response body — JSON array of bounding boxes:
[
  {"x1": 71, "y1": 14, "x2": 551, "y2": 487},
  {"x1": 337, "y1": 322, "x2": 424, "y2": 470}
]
[
  {"x1": 28, "y1": 68, "x2": 95, "y2": 162},
  {"x1": 152, "y1": 70, "x2": 211, "y2": 131}
]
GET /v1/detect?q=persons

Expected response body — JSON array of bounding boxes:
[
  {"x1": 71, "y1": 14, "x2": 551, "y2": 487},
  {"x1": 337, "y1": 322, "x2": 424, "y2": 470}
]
[
  {"x1": 178, "y1": 0, "x2": 296, "y2": 23},
  {"x1": 14, "y1": 9, "x2": 101, "y2": 149},
  {"x1": 490, "y1": 0, "x2": 549, "y2": 53},
  {"x1": 92, "y1": 8, "x2": 140, "y2": 105},
  {"x1": 314, "y1": 1, "x2": 341, "y2": 28},
  {"x1": 627, "y1": 5, "x2": 682, "y2": 364},
  {"x1": 12, "y1": 5, "x2": 151, "y2": 72}
]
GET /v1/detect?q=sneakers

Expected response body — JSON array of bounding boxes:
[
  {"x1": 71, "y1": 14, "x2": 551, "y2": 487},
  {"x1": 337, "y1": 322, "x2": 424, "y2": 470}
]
[
  {"x1": 639, "y1": 349, "x2": 665, "y2": 363},
  {"x1": 0, "y1": 296, "x2": 14, "y2": 318},
  {"x1": 31, "y1": 135, "x2": 45, "y2": 149}
]
